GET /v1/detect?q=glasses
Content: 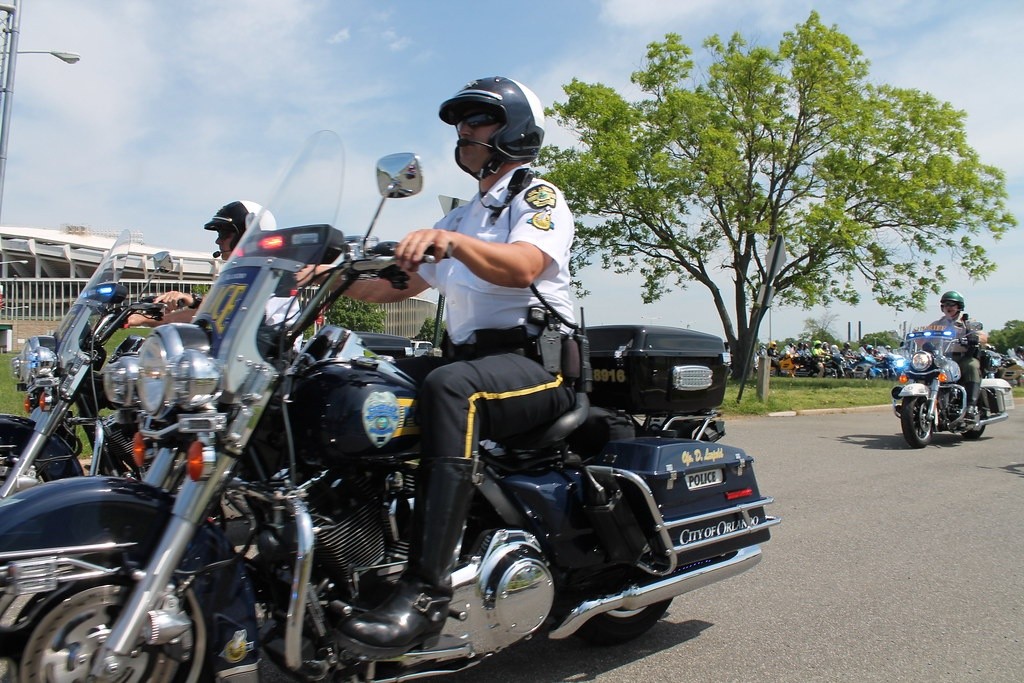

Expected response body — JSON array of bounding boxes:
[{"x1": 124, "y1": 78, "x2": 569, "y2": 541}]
[
  {"x1": 456, "y1": 112, "x2": 504, "y2": 135},
  {"x1": 944, "y1": 302, "x2": 957, "y2": 307},
  {"x1": 219, "y1": 228, "x2": 236, "y2": 240}
]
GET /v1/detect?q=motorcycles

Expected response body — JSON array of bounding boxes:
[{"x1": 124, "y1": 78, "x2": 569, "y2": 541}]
[
  {"x1": 724, "y1": 345, "x2": 1024, "y2": 387},
  {"x1": 0, "y1": 129, "x2": 783, "y2": 683},
  {"x1": 0, "y1": 230, "x2": 418, "y2": 517},
  {"x1": 891, "y1": 322, "x2": 1016, "y2": 449}
]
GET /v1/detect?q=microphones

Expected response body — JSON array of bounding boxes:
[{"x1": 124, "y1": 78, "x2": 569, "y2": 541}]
[
  {"x1": 457, "y1": 138, "x2": 494, "y2": 152},
  {"x1": 213, "y1": 250, "x2": 232, "y2": 258},
  {"x1": 947, "y1": 309, "x2": 963, "y2": 313}
]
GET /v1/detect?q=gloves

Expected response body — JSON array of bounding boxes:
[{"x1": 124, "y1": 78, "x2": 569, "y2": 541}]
[
  {"x1": 900, "y1": 341, "x2": 906, "y2": 348},
  {"x1": 966, "y1": 335, "x2": 978, "y2": 345}
]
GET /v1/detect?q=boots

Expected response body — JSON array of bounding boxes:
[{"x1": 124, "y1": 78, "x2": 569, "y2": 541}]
[
  {"x1": 964, "y1": 381, "x2": 980, "y2": 415},
  {"x1": 335, "y1": 457, "x2": 480, "y2": 659}
]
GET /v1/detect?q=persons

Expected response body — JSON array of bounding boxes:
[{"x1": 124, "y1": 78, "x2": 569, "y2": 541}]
[
  {"x1": 292, "y1": 75, "x2": 580, "y2": 660},
  {"x1": 1014, "y1": 345, "x2": 1024, "y2": 358},
  {"x1": 758, "y1": 340, "x2": 894, "y2": 378},
  {"x1": 911, "y1": 289, "x2": 988, "y2": 417},
  {"x1": 124, "y1": 199, "x2": 303, "y2": 367}
]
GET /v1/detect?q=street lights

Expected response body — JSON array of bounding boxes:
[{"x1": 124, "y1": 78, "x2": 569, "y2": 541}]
[{"x1": 0, "y1": 1, "x2": 82, "y2": 214}]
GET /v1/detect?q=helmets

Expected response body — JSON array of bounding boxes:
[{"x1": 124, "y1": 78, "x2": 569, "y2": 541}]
[
  {"x1": 439, "y1": 76, "x2": 545, "y2": 161},
  {"x1": 760, "y1": 339, "x2": 891, "y2": 351},
  {"x1": 1017, "y1": 346, "x2": 1024, "y2": 352},
  {"x1": 204, "y1": 200, "x2": 277, "y2": 255},
  {"x1": 940, "y1": 291, "x2": 964, "y2": 312}
]
